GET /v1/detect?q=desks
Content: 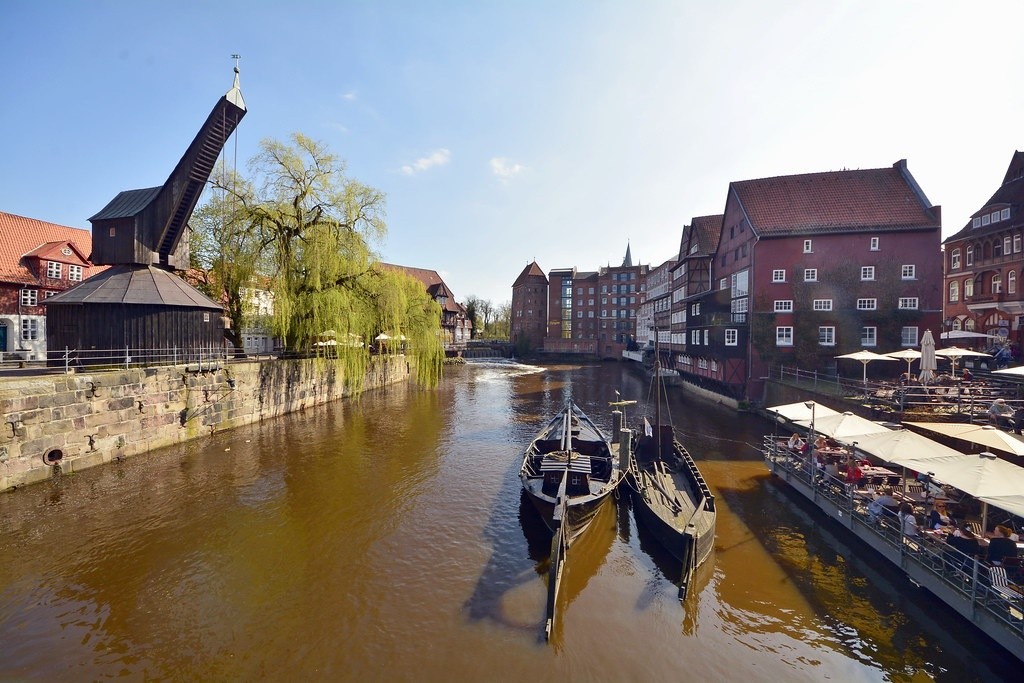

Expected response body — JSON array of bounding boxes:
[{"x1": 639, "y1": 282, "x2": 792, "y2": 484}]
[
  {"x1": 846, "y1": 489, "x2": 959, "y2": 505},
  {"x1": 790, "y1": 445, "x2": 853, "y2": 458},
  {"x1": 923, "y1": 528, "x2": 1024, "y2": 550},
  {"x1": 838, "y1": 466, "x2": 902, "y2": 479}
]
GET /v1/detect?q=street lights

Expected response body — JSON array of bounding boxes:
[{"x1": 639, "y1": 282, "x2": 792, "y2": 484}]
[{"x1": 944, "y1": 317, "x2": 952, "y2": 347}]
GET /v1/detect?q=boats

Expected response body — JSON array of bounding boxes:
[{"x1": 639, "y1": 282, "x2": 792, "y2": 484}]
[
  {"x1": 646, "y1": 326, "x2": 680, "y2": 386},
  {"x1": 520, "y1": 397, "x2": 613, "y2": 643},
  {"x1": 764, "y1": 399, "x2": 1024, "y2": 668},
  {"x1": 629, "y1": 321, "x2": 715, "y2": 603}
]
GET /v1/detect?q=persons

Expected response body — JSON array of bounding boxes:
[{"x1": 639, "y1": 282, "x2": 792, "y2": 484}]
[
  {"x1": 787, "y1": 432, "x2": 1018, "y2": 591},
  {"x1": 989, "y1": 398, "x2": 1024, "y2": 435},
  {"x1": 899, "y1": 337, "x2": 1019, "y2": 388}
]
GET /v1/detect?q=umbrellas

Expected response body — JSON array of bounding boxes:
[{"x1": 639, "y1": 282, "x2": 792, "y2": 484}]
[
  {"x1": 834, "y1": 329, "x2": 1000, "y2": 387},
  {"x1": 763, "y1": 400, "x2": 1024, "y2": 518},
  {"x1": 314, "y1": 331, "x2": 404, "y2": 347}
]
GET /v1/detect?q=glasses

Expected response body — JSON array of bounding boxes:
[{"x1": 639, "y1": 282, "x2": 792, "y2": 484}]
[{"x1": 938, "y1": 506, "x2": 945, "y2": 507}]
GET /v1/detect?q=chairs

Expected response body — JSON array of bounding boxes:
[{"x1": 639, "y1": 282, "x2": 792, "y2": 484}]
[{"x1": 776, "y1": 440, "x2": 1024, "y2": 610}]
